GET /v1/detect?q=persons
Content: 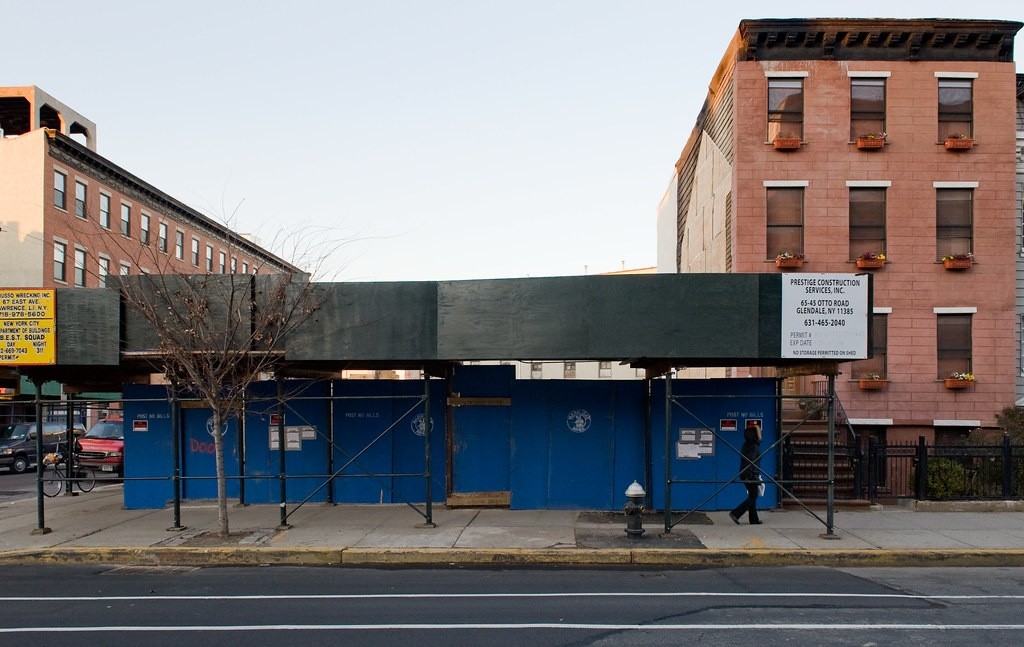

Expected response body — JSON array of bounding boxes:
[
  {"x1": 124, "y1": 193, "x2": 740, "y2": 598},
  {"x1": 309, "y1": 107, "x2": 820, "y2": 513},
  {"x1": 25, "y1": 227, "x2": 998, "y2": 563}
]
[{"x1": 728, "y1": 425, "x2": 763, "y2": 524}]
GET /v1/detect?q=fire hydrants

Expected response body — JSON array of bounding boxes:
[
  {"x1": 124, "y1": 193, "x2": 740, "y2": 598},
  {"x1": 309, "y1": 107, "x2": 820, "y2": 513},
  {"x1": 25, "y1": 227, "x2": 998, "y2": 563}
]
[{"x1": 623, "y1": 480, "x2": 648, "y2": 540}]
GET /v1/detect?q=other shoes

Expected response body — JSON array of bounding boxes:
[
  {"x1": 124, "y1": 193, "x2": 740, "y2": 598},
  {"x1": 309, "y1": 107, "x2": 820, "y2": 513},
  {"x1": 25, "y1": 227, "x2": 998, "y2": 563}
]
[
  {"x1": 729, "y1": 512, "x2": 741, "y2": 525},
  {"x1": 750, "y1": 520, "x2": 763, "y2": 525}
]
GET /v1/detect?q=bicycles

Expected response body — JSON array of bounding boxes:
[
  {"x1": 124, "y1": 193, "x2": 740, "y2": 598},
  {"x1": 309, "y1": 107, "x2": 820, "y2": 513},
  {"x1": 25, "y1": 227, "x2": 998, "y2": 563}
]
[{"x1": 44, "y1": 435, "x2": 96, "y2": 496}]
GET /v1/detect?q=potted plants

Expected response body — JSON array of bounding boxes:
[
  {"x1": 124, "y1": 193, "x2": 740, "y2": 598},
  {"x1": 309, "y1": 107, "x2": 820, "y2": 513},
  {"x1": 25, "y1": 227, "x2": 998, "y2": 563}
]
[
  {"x1": 795, "y1": 393, "x2": 823, "y2": 420},
  {"x1": 774, "y1": 132, "x2": 802, "y2": 148}
]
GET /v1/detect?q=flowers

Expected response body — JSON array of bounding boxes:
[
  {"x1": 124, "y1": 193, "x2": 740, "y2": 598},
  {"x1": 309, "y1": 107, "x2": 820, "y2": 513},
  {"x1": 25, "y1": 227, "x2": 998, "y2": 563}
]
[
  {"x1": 777, "y1": 252, "x2": 804, "y2": 259},
  {"x1": 947, "y1": 133, "x2": 967, "y2": 140},
  {"x1": 858, "y1": 132, "x2": 887, "y2": 140},
  {"x1": 862, "y1": 250, "x2": 886, "y2": 259},
  {"x1": 942, "y1": 252, "x2": 974, "y2": 261},
  {"x1": 950, "y1": 372, "x2": 975, "y2": 381},
  {"x1": 863, "y1": 373, "x2": 880, "y2": 379}
]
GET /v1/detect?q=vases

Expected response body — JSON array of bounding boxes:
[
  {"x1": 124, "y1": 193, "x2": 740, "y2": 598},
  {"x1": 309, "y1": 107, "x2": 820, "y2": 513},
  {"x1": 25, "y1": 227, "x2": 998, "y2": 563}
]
[
  {"x1": 945, "y1": 379, "x2": 970, "y2": 388},
  {"x1": 858, "y1": 380, "x2": 887, "y2": 388},
  {"x1": 856, "y1": 259, "x2": 884, "y2": 267},
  {"x1": 775, "y1": 258, "x2": 802, "y2": 267},
  {"x1": 857, "y1": 139, "x2": 884, "y2": 148},
  {"x1": 944, "y1": 139, "x2": 974, "y2": 148},
  {"x1": 944, "y1": 260, "x2": 972, "y2": 268}
]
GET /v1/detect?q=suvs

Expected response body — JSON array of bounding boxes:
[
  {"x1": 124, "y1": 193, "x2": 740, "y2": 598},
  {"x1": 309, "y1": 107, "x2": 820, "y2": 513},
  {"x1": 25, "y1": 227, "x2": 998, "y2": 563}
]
[
  {"x1": 0, "y1": 420, "x2": 86, "y2": 473},
  {"x1": 75, "y1": 416, "x2": 126, "y2": 479}
]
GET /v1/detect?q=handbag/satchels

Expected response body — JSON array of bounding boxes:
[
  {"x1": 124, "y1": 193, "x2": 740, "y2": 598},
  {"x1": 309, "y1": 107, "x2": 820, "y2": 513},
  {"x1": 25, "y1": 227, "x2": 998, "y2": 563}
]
[{"x1": 747, "y1": 474, "x2": 765, "y2": 497}]
